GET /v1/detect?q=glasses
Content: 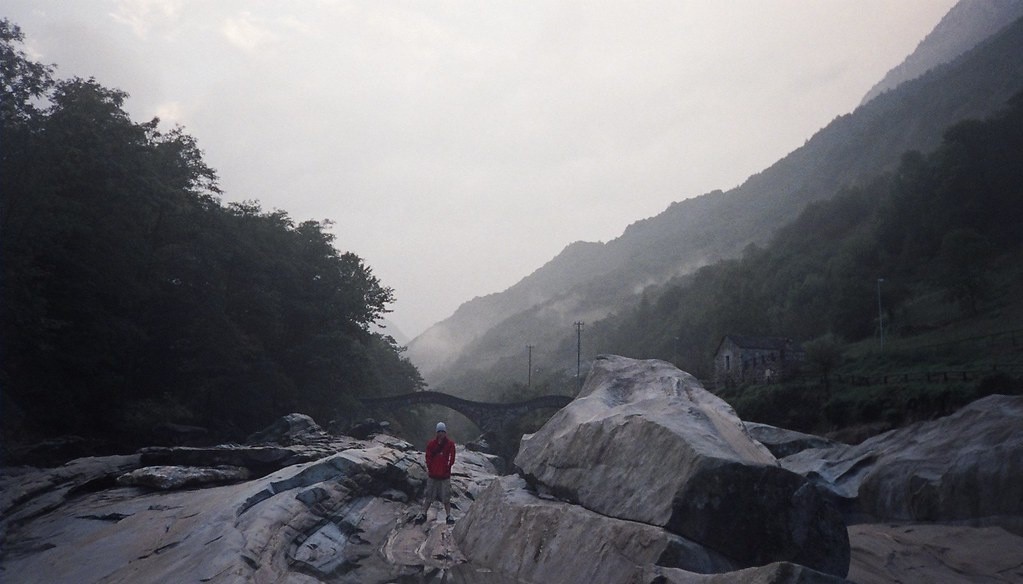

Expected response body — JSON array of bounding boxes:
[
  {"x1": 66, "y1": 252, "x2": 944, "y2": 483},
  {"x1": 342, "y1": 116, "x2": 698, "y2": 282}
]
[{"x1": 437, "y1": 430, "x2": 445, "y2": 433}]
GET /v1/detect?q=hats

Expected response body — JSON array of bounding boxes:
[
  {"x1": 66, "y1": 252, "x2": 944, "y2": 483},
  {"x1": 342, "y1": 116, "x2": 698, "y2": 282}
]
[{"x1": 436, "y1": 422, "x2": 447, "y2": 433}]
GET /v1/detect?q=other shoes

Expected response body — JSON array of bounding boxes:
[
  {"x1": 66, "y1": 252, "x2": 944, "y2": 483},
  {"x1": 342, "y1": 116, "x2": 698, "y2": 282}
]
[
  {"x1": 415, "y1": 513, "x2": 427, "y2": 524},
  {"x1": 446, "y1": 516, "x2": 455, "y2": 524}
]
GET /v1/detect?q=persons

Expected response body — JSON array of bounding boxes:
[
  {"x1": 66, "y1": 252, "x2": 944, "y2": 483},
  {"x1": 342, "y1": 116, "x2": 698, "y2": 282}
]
[{"x1": 415, "y1": 422, "x2": 455, "y2": 524}]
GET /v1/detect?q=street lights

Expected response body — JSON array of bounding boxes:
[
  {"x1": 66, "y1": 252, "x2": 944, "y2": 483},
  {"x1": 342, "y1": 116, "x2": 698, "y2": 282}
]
[{"x1": 877, "y1": 278, "x2": 885, "y2": 358}]
